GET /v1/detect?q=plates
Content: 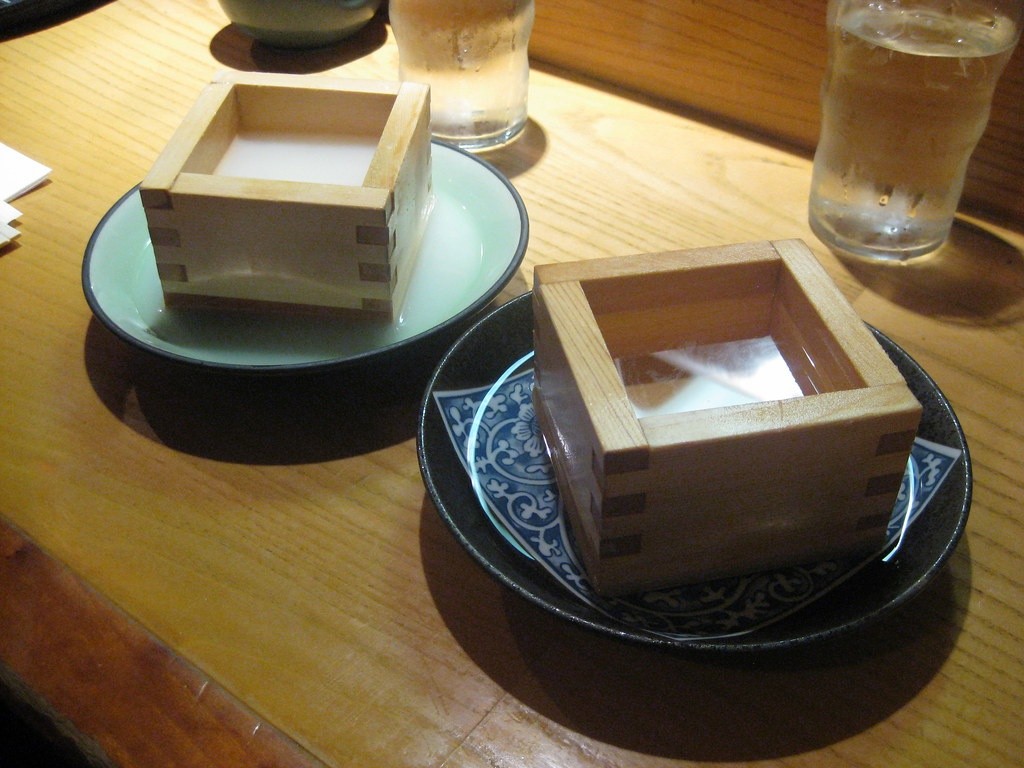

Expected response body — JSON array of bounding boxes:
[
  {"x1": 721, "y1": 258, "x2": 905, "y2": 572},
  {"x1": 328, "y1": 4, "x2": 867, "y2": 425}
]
[
  {"x1": 81, "y1": 140, "x2": 530, "y2": 371},
  {"x1": 415, "y1": 289, "x2": 974, "y2": 653}
]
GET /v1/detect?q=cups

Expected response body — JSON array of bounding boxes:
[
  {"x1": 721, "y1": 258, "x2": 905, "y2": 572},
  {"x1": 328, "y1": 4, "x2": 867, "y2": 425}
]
[
  {"x1": 386, "y1": 1, "x2": 535, "y2": 154},
  {"x1": 808, "y1": 1, "x2": 1023, "y2": 267}
]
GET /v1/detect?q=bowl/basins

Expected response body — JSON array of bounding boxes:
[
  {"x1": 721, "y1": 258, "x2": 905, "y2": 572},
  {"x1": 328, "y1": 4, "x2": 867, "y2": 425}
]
[{"x1": 212, "y1": 0, "x2": 384, "y2": 47}]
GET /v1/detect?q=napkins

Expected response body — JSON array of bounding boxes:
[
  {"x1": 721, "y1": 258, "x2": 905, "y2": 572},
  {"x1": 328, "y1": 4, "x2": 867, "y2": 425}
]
[{"x1": 0, "y1": 141, "x2": 53, "y2": 247}]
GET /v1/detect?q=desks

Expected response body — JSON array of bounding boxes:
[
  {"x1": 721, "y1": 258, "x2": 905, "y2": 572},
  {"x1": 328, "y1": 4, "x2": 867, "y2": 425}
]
[{"x1": 0, "y1": 0, "x2": 1023, "y2": 768}]
